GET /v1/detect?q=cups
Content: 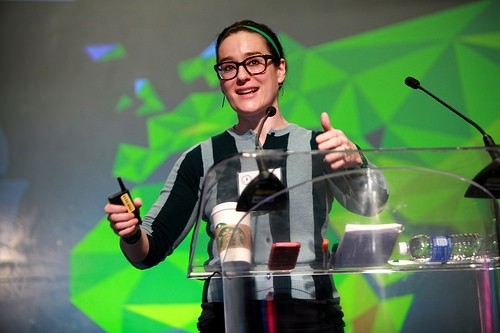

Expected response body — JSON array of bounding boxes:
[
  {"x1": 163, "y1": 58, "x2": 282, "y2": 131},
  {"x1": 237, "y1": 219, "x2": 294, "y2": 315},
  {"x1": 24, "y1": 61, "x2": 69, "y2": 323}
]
[{"x1": 211, "y1": 202, "x2": 251, "y2": 270}]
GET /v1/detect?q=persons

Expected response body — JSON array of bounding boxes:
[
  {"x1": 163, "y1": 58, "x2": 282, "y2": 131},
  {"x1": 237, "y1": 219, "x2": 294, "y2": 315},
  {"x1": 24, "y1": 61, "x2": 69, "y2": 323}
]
[{"x1": 104, "y1": 19, "x2": 389, "y2": 333}]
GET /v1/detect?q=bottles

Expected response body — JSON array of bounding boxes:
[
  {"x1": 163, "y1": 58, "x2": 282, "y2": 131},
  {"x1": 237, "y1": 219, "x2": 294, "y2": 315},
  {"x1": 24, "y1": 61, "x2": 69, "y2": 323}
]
[{"x1": 399, "y1": 233, "x2": 485, "y2": 262}]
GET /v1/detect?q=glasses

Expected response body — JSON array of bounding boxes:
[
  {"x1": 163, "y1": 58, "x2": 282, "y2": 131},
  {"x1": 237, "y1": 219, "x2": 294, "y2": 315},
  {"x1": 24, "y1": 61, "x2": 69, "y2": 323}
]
[{"x1": 212, "y1": 52, "x2": 282, "y2": 82}]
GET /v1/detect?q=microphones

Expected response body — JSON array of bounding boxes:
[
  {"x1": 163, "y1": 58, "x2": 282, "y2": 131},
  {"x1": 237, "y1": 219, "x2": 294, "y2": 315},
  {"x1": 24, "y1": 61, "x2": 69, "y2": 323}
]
[
  {"x1": 236, "y1": 106, "x2": 288, "y2": 212},
  {"x1": 405, "y1": 77, "x2": 500, "y2": 198}
]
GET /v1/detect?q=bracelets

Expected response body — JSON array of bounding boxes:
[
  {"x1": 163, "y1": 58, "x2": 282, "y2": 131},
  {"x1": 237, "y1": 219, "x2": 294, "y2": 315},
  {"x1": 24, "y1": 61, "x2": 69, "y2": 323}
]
[
  {"x1": 347, "y1": 143, "x2": 368, "y2": 178},
  {"x1": 123, "y1": 229, "x2": 142, "y2": 244}
]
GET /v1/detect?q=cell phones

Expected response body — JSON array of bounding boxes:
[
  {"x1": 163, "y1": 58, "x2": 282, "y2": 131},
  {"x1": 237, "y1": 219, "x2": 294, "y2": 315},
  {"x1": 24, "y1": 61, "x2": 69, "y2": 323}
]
[{"x1": 268, "y1": 242, "x2": 300, "y2": 270}]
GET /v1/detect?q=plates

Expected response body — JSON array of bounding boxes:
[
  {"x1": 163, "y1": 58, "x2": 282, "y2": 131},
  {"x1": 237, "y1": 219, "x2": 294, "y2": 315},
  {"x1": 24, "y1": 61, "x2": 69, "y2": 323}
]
[{"x1": 388, "y1": 257, "x2": 499, "y2": 265}]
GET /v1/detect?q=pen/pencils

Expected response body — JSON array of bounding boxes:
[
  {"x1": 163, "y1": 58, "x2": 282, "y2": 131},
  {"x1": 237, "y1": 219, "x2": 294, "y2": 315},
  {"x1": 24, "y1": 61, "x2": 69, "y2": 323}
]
[
  {"x1": 327, "y1": 243, "x2": 338, "y2": 267},
  {"x1": 322, "y1": 238, "x2": 329, "y2": 268}
]
